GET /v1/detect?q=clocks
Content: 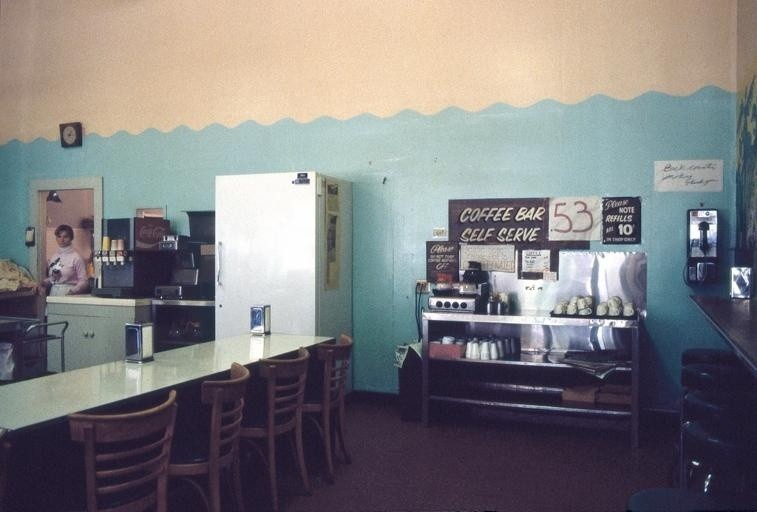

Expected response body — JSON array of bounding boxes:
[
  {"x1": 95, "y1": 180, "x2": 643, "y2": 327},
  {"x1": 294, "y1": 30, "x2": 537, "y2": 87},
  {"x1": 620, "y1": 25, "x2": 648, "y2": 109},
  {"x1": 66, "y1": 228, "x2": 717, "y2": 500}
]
[{"x1": 59, "y1": 123, "x2": 82, "y2": 147}]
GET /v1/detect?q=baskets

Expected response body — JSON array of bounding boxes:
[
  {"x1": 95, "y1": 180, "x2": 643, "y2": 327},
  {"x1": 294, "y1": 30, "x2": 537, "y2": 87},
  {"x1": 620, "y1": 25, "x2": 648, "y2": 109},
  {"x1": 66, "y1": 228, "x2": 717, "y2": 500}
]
[{"x1": 428, "y1": 343, "x2": 467, "y2": 359}]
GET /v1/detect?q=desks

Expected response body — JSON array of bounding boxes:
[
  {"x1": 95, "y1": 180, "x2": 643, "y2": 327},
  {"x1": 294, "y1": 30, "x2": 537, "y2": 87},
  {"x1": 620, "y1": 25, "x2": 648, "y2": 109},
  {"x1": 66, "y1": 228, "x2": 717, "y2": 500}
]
[
  {"x1": 1, "y1": 338, "x2": 373, "y2": 440},
  {"x1": 690, "y1": 294, "x2": 757, "y2": 377}
]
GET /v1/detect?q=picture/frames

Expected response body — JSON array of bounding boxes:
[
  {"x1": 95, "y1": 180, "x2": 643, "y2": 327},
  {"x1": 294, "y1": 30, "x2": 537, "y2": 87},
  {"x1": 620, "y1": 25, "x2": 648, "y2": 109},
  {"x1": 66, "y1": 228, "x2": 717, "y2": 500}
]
[{"x1": 731, "y1": 267, "x2": 752, "y2": 299}]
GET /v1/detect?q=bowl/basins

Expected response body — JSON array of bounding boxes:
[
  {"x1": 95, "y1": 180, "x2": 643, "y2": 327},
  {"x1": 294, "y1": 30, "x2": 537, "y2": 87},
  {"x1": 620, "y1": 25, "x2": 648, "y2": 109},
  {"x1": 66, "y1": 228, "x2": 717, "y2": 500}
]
[{"x1": 429, "y1": 336, "x2": 465, "y2": 345}]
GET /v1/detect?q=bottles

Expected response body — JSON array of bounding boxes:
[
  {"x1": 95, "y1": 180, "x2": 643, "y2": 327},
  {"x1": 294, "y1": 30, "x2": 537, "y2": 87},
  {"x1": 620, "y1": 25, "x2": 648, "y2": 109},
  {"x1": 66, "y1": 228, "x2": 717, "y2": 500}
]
[
  {"x1": 461, "y1": 260, "x2": 485, "y2": 296},
  {"x1": 431, "y1": 272, "x2": 456, "y2": 297},
  {"x1": 465, "y1": 335, "x2": 516, "y2": 362}
]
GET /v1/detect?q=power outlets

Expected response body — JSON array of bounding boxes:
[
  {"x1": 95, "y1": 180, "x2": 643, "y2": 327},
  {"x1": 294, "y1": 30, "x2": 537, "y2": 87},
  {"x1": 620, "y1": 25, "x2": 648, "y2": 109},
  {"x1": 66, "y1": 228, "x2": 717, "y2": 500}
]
[{"x1": 416, "y1": 282, "x2": 430, "y2": 293}]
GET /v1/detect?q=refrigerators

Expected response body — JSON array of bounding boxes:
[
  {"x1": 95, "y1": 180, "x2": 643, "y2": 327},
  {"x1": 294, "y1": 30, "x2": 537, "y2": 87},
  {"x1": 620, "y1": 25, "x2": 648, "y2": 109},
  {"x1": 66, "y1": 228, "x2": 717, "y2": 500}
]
[{"x1": 214, "y1": 172, "x2": 354, "y2": 402}]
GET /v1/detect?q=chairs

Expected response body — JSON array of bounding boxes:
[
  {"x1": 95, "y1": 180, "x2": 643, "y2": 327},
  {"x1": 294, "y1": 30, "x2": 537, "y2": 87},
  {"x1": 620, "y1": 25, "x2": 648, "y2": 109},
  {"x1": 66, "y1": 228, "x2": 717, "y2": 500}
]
[
  {"x1": 303, "y1": 334, "x2": 354, "y2": 484},
  {"x1": 241, "y1": 347, "x2": 314, "y2": 511},
  {"x1": 171, "y1": 363, "x2": 251, "y2": 512},
  {"x1": 68, "y1": 390, "x2": 178, "y2": 512},
  {"x1": 683, "y1": 348, "x2": 756, "y2": 491}
]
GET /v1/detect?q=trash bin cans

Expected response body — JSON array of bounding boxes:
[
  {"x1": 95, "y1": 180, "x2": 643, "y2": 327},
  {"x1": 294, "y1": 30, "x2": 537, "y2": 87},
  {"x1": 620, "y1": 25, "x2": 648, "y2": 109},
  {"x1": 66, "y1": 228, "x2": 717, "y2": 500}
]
[{"x1": 398, "y1": 339, "x2": 422, "y2": 422}]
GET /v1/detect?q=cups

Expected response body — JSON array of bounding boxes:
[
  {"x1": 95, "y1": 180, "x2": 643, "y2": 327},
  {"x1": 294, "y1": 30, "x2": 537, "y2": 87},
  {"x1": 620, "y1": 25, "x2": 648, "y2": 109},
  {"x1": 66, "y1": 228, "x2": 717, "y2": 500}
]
[
  {"x1": 551, "y1": 295, "x2": 634, "y2": 318},
  {"x1": 485, "y1": 301, "x2": 504, "y2": 315},
  {"x1": 111, "y1": 237, "x2": 124, "y2": 251},
  {"x1": 101, "y1": 235, "x2": 111, "y2": 251}
]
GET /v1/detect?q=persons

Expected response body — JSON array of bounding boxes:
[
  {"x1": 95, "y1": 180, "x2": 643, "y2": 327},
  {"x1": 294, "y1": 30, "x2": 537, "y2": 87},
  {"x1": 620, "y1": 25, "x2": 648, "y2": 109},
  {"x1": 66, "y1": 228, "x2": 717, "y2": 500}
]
[{"x1": 41, "y1": 224, "x2": 89, "y2": 297}]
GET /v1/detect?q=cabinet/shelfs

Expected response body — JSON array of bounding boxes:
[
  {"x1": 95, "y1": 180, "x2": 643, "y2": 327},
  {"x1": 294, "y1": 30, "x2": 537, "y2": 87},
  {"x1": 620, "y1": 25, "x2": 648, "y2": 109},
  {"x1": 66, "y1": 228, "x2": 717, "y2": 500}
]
[
  {"x1": 46, "y1": 294, "x2": 151, "y2": 372},
  {"x1": 423, "y1": 312, "x2": 642, "y2": 448}
]
